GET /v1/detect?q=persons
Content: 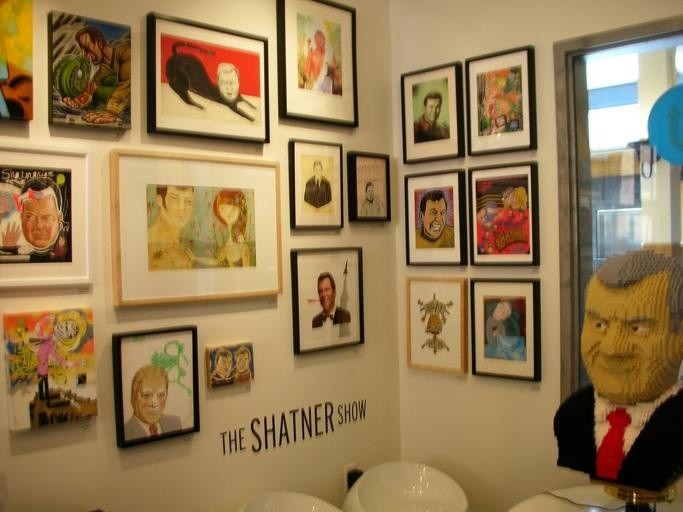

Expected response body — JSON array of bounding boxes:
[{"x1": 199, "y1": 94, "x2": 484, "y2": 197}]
[
  {"x1": 313, "y1": 273, "x2": 351, "y2": 330},
  {"x1": 552, "y1": 246, "x2": 683, "y2": 489},
  {"x1": 413, "y1": 89, "x2": 450, "y2": 144},
  {"x1": 415, "y1": 188, "x2": 455, "y2": 249}
]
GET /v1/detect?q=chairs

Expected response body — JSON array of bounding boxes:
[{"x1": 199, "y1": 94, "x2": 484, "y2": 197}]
[
  {"x1": 340, "y1": 460, "x2": 471, "y2": 511},
  {"x1": 234, "y1": 489, "x2": 342, "y2": 512}
]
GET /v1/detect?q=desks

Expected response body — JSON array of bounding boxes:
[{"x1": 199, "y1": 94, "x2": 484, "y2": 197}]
[{"x1": 503, "y1": 483, "x2": 683, "y2": 512}]
